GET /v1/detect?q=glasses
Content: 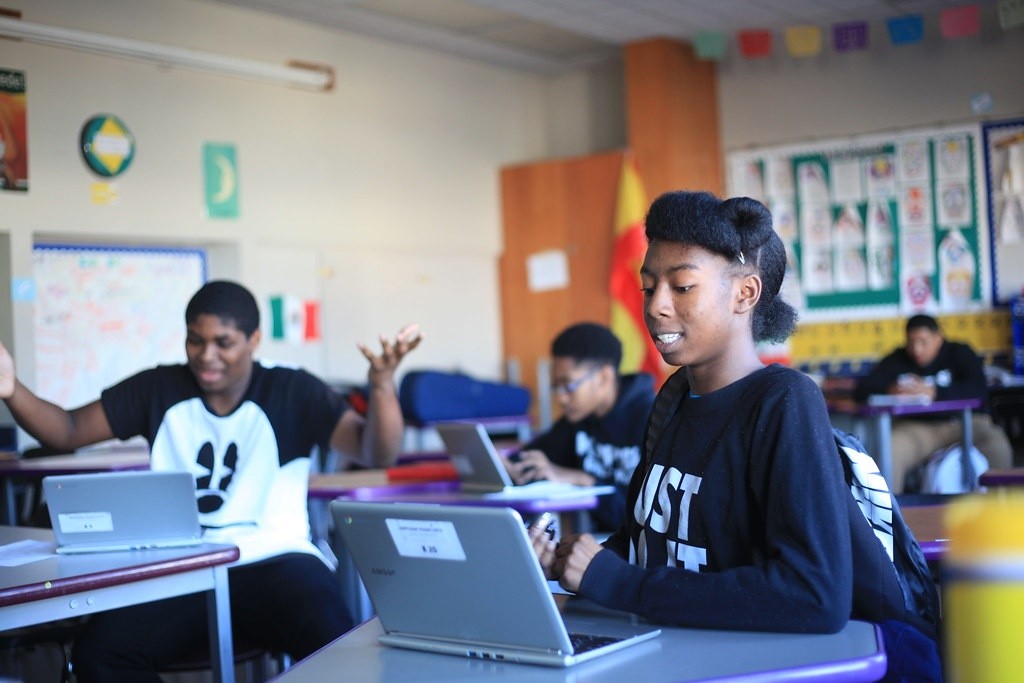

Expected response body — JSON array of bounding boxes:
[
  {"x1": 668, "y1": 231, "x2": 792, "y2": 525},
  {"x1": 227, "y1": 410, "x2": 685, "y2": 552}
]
[{"x1": 551, "y1": 366, "x2": 601, "y2": 395}]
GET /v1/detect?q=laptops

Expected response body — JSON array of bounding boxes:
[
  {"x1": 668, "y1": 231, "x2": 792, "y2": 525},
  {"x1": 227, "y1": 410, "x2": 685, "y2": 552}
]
[
  {"x1": 436, "y1": 424, "x2": 515, "y2": 491},
  {"x1": 332, "y1": 500, "x2": 660, "y2": 666},
  {"x1": 41, "y1": 471, "x2": 204, "y2": 553}
]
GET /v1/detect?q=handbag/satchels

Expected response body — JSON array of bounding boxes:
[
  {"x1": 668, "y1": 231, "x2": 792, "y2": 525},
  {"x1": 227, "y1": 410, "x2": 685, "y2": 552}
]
[{"x1": 918, "y1": 441, "x2": 989, "y2": 494}]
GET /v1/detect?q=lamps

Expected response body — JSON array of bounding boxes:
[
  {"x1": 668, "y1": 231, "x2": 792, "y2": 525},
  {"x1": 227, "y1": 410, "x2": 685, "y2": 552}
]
[{"x1": 1, "y1": 9, "x2": 336, "y2": 93}]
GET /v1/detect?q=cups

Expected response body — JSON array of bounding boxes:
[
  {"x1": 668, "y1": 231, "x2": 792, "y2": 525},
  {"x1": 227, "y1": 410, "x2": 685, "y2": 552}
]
[{"x1": 944, "y1": 490, "x2": 1024, "y2": 683}]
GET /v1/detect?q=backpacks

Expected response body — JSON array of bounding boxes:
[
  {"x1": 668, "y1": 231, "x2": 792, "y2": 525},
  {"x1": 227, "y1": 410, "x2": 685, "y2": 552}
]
[{"x1": 645, "y1": 365, "x2": 942, "y2": 624}]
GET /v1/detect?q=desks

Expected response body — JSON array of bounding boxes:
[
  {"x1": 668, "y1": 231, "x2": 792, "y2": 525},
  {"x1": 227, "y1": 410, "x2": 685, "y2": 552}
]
[
  {"x1": 0, "y1": 525, "x2": 241, "y2": 683},
  {"x1": 339, "y1": 479, "x2": 598, "y2": 630},
  {"x1": 826, "y1": 394, "x2": 981, "y2": 506},
  {"x1": 268, "y1": 604, "x2": 887, "y2": 683},
  {"x1": 0, "y1": 445, "x2": 151, "y2": 526}
]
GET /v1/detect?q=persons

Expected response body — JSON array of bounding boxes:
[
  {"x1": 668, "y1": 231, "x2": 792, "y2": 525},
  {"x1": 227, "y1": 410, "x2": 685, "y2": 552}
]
[
  {"x1": 497, "y1": 320, "x2": 657, "y2": 567},
  {"x1": 850, "y1": 314, "x2": 986, "y2": 423},
  {"x1": 0, "y1": 280, "x2": 423, "y2": 683},
  {"x1": 524, "y1": 191, "x2": 945, "y2": 683}
]
